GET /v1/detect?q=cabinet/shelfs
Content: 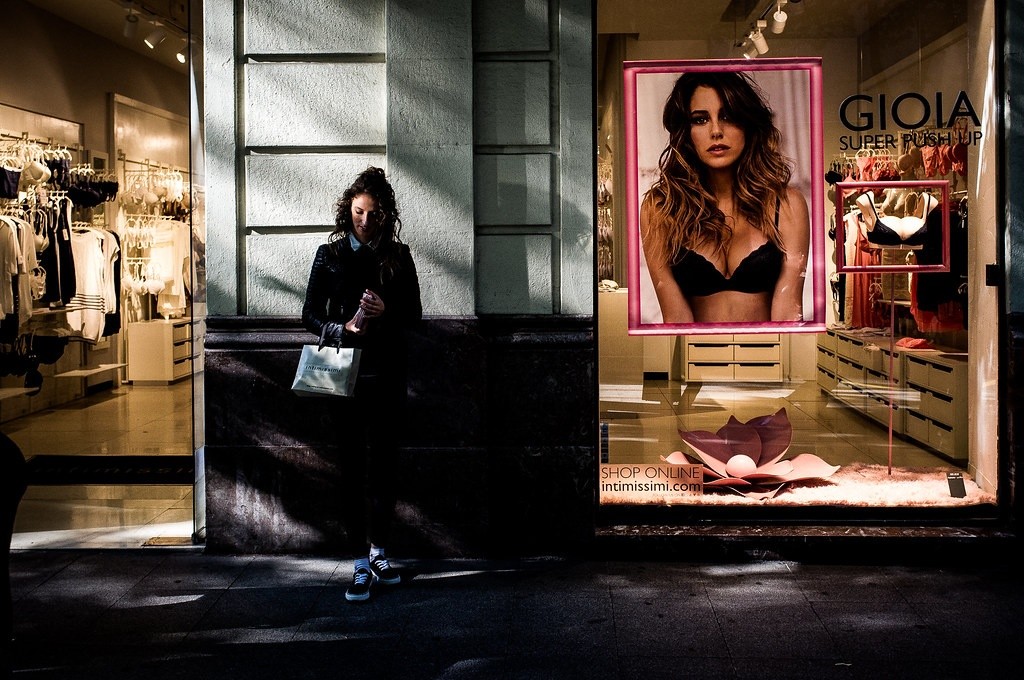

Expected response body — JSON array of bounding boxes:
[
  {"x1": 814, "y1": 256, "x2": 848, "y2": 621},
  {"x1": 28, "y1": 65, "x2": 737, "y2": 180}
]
[
  {"x1": 126, "y1": 318, "x2": 205, "y2": 386},
  {"x1": 816, "y1": 327, "x2": 969, "y2": 459},
  {"x1": 681, "y1": 334, "x2": 783, "y2": 383}
]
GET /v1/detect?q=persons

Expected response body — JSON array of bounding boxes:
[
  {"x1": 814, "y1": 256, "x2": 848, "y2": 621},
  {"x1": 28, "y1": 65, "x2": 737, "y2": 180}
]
[
  {"x1": 640, "y1": 72, "x2": 810, "y2": 324},
  {"x1": 301, "y1": 167, "x2": 424, "y2": 602}
]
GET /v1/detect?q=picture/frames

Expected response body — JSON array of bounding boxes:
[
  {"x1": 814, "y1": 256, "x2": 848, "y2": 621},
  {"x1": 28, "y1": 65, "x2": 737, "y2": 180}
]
[{"x1": 623, "y1": 57, "x2": 827, "y2": 335}]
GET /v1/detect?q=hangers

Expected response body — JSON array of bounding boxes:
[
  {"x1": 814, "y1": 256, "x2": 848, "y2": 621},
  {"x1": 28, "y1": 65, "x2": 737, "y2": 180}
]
[
  {"x1": 0, "y1": 138, "x2": 187, "y2": 281},
  {"x1": 830, "y1": 128, "x2": 967, "y2": 178}
]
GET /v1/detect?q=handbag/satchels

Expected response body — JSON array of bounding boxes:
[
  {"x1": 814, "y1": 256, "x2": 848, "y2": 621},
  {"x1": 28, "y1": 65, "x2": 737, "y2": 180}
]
[{"x1": 291, "y1": 321, "x2": 362, "y2": 398}]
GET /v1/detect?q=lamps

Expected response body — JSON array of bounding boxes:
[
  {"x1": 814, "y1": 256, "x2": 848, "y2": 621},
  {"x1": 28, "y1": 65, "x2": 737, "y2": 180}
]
[
  {"x1": 122, "y1": 0, "x2": 196, "y2": 64},
  {"x1": 744, "y1": 0, "x2": 787, "y2": 61}
]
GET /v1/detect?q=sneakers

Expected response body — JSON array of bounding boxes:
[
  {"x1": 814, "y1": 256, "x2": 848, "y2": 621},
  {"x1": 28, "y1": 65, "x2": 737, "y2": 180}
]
[
  {"x1": 368, "y1": 554, "x2": 401, "y2": 585},
  {"x1": 345, "y1": 568, "x2": 372, "y2": 600}
]
